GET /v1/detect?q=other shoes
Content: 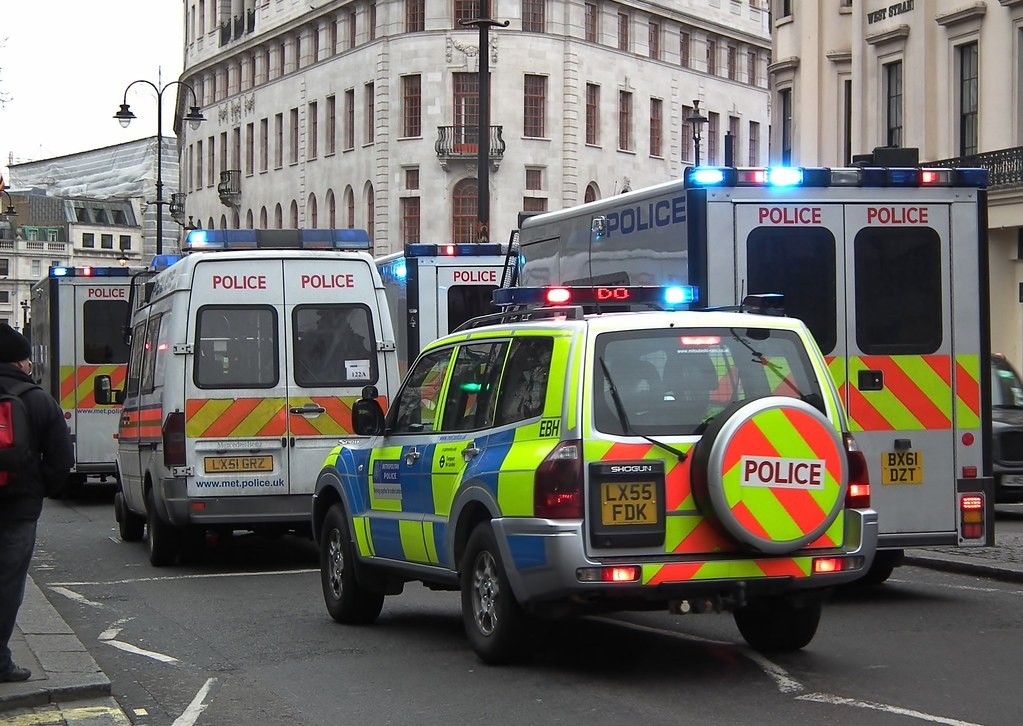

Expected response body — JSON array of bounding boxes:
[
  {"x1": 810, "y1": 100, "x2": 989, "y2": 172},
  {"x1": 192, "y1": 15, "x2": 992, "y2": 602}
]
[{"x1": 0, "y1": 661, "x2": 31, "y2": 683}]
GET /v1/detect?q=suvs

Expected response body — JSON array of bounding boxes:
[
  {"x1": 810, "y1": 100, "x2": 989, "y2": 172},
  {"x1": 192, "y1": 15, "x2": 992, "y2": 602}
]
[{"x1": 311, "y1": 284, "x2": 878, "y2": 659}]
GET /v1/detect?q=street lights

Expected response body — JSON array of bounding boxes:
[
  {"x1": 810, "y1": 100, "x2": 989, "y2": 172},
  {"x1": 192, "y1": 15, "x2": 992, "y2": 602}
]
[
  {"x1": 685, "y1": 101, "x2": 706, "y2": 164},
  {"x1": 114, "y1": 80, "x2": 208, "y2": 257},
  {"x1": 22, "y1": 305, "x2": 30, "y2": 333}
]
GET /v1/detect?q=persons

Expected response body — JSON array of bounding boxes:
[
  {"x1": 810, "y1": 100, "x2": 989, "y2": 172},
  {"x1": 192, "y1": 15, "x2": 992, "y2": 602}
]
[{"x1": 0, "y1": 322, "x2": 74, "y2": 684}]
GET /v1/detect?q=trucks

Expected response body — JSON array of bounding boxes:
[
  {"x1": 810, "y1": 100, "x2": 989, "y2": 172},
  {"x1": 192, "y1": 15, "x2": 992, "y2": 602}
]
[
  {"x1": 29, "y1": 266, "x2": 161, "y2": 496},
  {"x1": 493, "y1": 167, "x2": 997, "y2": 596},
  {"x1": 375, "y1": 244, "x2": 519, "y2": 420}
]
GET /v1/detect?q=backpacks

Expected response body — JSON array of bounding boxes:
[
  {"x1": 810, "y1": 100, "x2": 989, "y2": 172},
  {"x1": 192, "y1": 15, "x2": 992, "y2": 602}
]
[{"x1": 0, "y1": 382, "x2": 42, "y2": 491}]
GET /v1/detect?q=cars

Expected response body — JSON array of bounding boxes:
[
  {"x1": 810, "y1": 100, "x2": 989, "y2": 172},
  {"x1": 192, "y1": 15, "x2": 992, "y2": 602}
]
[{"x1": 990, "y1": 354, "x2": 1022, "y2": 504}]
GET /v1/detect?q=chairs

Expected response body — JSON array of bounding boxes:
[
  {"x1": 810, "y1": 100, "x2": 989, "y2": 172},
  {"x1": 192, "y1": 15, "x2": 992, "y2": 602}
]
[{"x1": 613, "y1": 350, "x2": 719, "y2": 425}]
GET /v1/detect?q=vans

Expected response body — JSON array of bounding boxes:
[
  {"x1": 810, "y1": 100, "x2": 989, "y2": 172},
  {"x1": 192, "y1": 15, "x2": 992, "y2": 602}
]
[{"x1": 95, "y1": 229, "x2": 403, "y2": 569}]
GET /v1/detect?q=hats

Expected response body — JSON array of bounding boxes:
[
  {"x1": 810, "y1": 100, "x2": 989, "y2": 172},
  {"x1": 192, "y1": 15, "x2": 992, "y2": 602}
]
[{"x1": 0, "y1": 323, "x2": 32, "y2": 364}]
